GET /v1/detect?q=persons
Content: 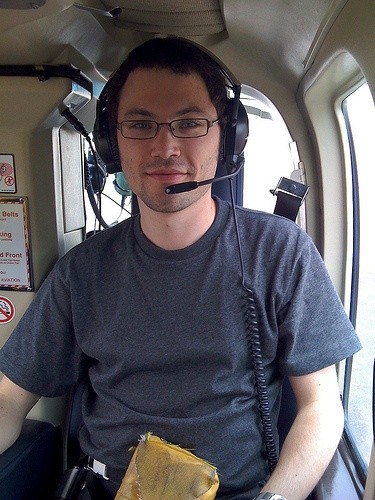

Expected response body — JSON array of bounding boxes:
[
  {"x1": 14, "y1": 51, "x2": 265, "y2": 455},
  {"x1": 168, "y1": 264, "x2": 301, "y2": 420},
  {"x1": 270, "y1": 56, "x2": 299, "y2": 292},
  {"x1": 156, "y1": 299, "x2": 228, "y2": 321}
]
[{"x1": 0, "y1": 35, "x2": 362, "y2": 500}]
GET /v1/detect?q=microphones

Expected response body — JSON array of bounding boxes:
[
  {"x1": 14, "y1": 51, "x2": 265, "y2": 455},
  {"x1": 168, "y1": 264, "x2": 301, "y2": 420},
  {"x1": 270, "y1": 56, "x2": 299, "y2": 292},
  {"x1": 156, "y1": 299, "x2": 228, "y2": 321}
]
[{"x1": 165, "y1": 163, "x2": 244, "y2": 194}]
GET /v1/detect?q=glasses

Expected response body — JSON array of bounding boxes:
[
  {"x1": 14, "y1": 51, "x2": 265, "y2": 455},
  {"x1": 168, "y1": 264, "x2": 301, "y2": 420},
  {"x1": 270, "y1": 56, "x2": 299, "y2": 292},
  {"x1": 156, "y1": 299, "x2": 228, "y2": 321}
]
[{"x1": 117, "y1": 118, "x2": 221, "y2": 140}]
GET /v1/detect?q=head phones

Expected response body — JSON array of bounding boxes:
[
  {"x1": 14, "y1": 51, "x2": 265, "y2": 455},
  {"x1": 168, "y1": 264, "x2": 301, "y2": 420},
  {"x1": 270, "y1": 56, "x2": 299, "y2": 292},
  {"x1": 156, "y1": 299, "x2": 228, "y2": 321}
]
[{"x1": 93, "y1": 36, "x2": 249, "y2": 174}]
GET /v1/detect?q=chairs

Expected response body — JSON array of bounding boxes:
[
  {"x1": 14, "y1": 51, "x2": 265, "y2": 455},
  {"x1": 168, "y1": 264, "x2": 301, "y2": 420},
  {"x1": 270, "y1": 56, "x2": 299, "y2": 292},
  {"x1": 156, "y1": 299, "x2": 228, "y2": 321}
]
[{"x1": 0, "y1": 152, "x2": 361, "y2": 500}]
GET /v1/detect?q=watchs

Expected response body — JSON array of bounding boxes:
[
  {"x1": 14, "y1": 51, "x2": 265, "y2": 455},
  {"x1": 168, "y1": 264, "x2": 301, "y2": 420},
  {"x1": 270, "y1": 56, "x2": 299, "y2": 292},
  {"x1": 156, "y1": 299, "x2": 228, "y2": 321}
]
[{"x1": 253, "y1": 492, "x2": 288, "y2": 500}]
